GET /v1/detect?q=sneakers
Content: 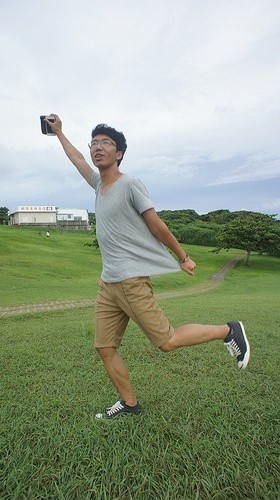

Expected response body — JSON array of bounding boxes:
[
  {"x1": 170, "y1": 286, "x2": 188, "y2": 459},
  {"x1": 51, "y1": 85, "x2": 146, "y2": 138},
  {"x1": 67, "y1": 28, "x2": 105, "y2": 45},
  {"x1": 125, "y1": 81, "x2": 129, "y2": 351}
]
[
  {"x1": 95, "y1": 400, "x2": 142, "y2": 420},
  {"x1": 223, "y1": 321, "x2": 250, "y2": 370}
]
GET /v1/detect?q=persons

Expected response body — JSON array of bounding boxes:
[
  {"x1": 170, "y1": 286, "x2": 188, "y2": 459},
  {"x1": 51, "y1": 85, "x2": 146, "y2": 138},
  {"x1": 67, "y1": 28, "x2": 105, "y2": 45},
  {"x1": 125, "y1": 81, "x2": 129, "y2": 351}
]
[
  {"x1": 44, "y1": 114, "x2": 251, "y2": 420},
  {"x1": 47, "y1": 231, "x2": 50, "y2": 239}
]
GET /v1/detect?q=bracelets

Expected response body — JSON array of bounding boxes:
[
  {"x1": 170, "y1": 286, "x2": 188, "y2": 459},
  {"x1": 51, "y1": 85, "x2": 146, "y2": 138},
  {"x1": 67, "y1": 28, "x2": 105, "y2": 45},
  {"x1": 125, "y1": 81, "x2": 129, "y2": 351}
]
[{"x1": 179, "y1": 254, "x2": 190, "y2": 263}]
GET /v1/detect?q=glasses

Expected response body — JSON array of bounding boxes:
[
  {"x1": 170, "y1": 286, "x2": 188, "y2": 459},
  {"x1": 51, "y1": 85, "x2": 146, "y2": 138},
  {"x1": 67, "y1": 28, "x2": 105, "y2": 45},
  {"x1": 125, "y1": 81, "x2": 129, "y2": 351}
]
[{"x1": 88, "y1": 138, "x2": 117, "y2": 148}]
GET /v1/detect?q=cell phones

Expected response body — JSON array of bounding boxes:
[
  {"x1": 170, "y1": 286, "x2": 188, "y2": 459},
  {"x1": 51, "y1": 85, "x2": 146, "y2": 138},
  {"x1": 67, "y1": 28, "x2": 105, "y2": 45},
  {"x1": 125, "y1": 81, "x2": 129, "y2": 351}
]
[{"x1": 45, "y1": 116, "x2": 57, "y2": 136}]
